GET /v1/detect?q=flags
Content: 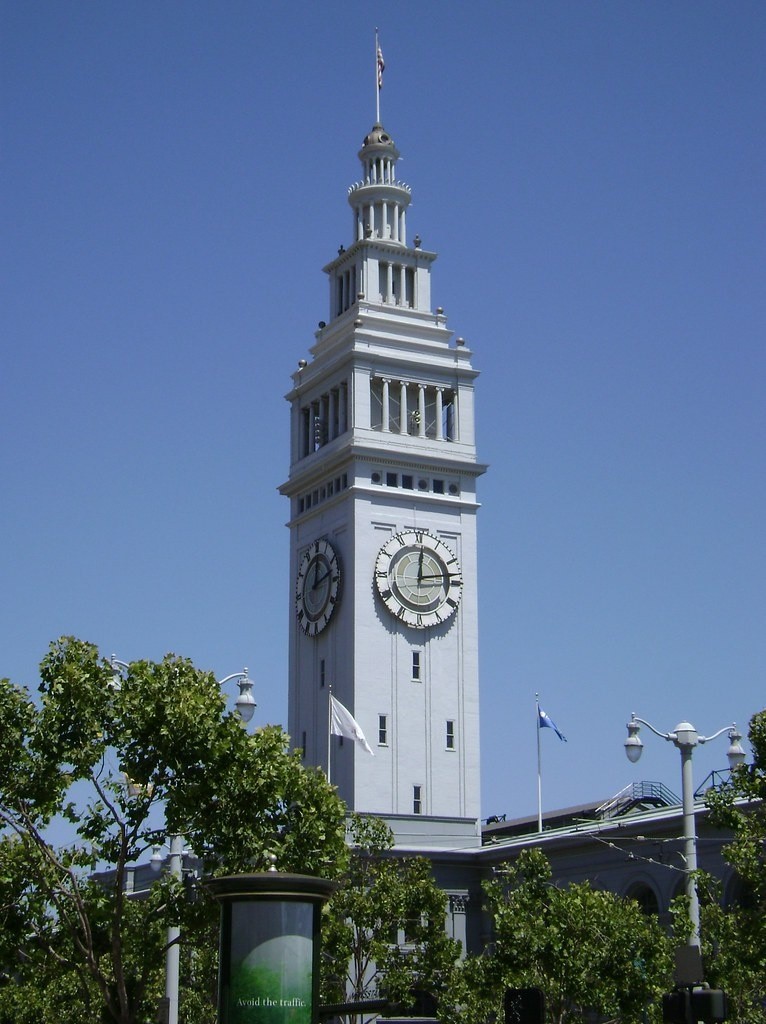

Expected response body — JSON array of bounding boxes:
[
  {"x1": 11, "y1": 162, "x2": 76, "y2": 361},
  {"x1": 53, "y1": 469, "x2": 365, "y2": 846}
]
[
  {"x1": 329, "y1": 695, "x2": 375, "y2": 758},
  {"x1": 538, "y1": 702, "x2": 569, "y2": 743}
]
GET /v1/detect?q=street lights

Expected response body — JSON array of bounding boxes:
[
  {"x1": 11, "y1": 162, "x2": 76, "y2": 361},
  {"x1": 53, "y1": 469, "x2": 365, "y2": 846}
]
[
  {"x1": 625, "y1": 712, "x2": 747, "y2": 1024},
  {"x1": 109, "y1": 659, "x2": 258, "y2": 1024}
]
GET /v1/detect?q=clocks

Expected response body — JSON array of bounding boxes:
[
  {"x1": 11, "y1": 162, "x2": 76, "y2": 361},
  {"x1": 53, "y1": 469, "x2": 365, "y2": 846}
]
[
  {"x1": 376, "y1": 529, "x2": 463, "y2": 627},
  {"x1": 297, "y1": 538, "x2": 340, "y2": 637}
]
